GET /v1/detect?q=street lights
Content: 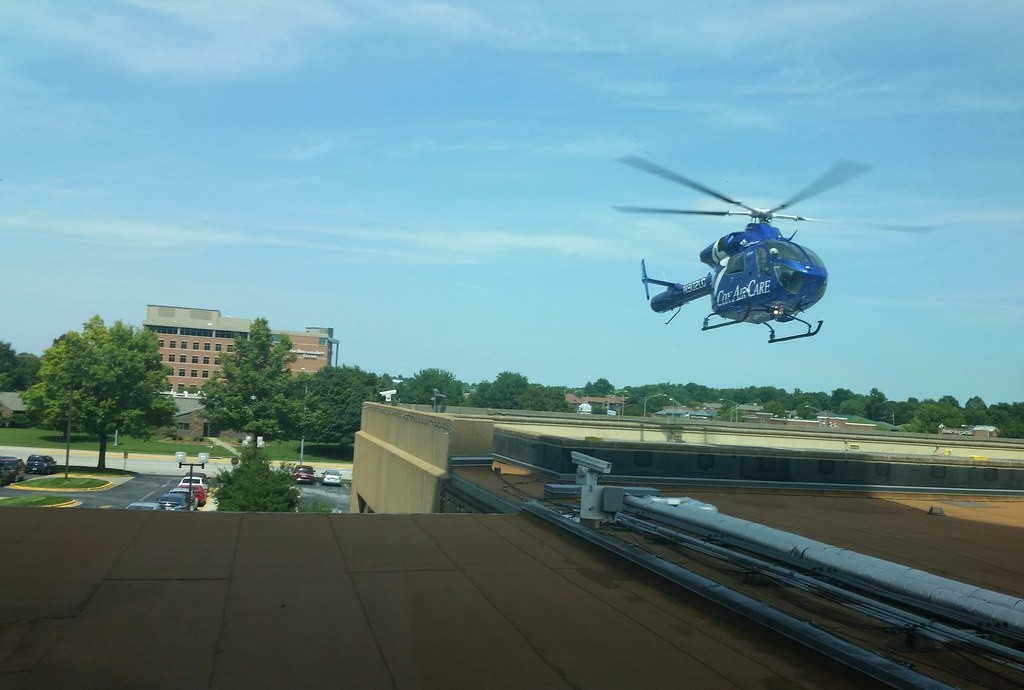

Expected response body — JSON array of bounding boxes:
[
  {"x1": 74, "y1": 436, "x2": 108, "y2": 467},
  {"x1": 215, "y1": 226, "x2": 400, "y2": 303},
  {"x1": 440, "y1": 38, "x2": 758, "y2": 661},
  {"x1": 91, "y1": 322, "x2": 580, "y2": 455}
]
[
  {"x1": 719, "y1": 398, "x2": 739, "y2": 423},
  {"x1": 804, "y1": 405, "x2": 828, "y2": 424},
  {"x1": 607, "y1": 390, "x2": 626, "y2": 416},
  {"x1": 644, "y1": 393, "x2": 668, "y2": 418},
  {"x1": 669, "y1": 398, "x2": 684, "y2": 417}
]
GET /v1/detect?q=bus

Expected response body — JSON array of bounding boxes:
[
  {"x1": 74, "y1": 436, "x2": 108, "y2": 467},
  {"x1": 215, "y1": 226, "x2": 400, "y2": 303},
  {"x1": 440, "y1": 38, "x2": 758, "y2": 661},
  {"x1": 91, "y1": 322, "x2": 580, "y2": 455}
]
[{"x1": 125, "y1": 502, "x2": 166, "y2": 511}]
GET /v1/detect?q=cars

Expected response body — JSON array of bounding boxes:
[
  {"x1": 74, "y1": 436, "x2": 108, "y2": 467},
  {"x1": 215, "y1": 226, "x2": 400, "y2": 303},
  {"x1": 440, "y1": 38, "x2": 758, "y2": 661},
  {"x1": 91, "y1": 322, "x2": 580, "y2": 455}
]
[
  {"x1": 185, "y1": 472, "x2": 211, "y2": 484},
  {"x1": 25, "y1": 454, "x2": 59, "y2": 475},
  {"x1": 176, "y1": 476, "x2": 208, "y2": 490},
  {"x1": 321, "y1": 469, "x2": 342, "y2": 486},
  {"x1": 169, "y1": 488, "x2": 199, "y2": 511},
  {"x1": 178, "y1": 484, "x2": 206, "y2": 506},
  {"x1": 155, "y1": 493, "x2": 189, "y2": 511}
]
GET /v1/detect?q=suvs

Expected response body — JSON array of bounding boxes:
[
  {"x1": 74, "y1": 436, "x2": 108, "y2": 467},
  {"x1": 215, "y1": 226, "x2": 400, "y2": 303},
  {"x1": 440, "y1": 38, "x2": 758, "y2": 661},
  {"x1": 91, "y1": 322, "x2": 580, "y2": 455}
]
[
  {"x1": 0, "y1": 456, "x2": 26, "y2": 487},
  {"x1": 294, "y1": 465, "x2": 316, "y2": 484}
]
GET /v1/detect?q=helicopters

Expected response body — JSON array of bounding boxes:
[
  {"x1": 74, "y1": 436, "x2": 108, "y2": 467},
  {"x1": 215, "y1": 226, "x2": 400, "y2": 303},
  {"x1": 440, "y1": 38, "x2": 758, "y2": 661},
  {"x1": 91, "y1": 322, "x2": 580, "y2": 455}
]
[{"x1": 610, "y1": 152, "x2": 867, "y2": 343}]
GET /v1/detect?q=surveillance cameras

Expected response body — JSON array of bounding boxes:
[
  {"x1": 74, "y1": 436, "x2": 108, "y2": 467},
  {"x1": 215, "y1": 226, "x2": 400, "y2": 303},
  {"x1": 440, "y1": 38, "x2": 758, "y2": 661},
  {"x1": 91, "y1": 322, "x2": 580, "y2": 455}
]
[
  {"x1": 570, "y1": 451, "x2": 612, "y2": 474},
  {"x1": 379, "y1": 390, "x2": 397, "y2": 397}
]
